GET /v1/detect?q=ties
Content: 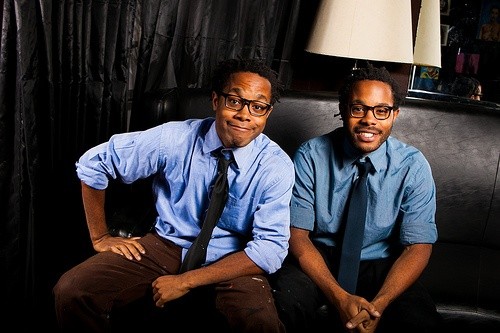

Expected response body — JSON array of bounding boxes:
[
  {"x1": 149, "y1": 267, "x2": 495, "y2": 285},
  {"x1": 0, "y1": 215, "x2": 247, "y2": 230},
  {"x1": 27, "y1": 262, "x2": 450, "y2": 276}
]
[
  {"x1": 179, "y1": 151, "x2": 235, "y2": 274},
  {"x1": 337, "y1": 160, "x2": 372, "y2": 296}
]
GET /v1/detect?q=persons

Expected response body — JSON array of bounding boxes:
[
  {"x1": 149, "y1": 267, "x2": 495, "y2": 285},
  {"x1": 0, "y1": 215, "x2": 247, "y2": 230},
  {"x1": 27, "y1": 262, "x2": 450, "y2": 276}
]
[
  {"x1": 446, "y1": 73, "x2": 483, "y2": 101},
  {"x1": 478, "y1": 5, "x2": 500, "y2": 42},
  {"x1": 51, "y1": 55, "x2": 295, "y2": 333},
  {"x1": 266, "y1": 62, "x2": 447, "y2": 333}
]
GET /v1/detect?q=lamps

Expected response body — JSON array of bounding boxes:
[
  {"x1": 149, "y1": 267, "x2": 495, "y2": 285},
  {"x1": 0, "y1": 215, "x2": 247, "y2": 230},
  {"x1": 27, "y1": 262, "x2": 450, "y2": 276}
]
[{"x1": 305, "y1": 0, "x2": 414, "y2": 80}]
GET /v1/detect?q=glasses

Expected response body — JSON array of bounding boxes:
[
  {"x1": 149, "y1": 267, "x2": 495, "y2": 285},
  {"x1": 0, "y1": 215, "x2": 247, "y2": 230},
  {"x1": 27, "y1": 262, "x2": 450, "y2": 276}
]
[
  {"x1": 218, "y1": 91, "x2": 271, "y2": 116},
  {"x1": 345, "y1": 103, "x2": 394, "y2": 120}
]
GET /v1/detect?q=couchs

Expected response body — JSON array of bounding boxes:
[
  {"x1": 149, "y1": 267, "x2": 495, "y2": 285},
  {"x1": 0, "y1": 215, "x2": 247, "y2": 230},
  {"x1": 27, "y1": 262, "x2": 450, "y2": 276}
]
[{"x1": 110, "y1": 87, "x2": 500, "y2": 333}]
